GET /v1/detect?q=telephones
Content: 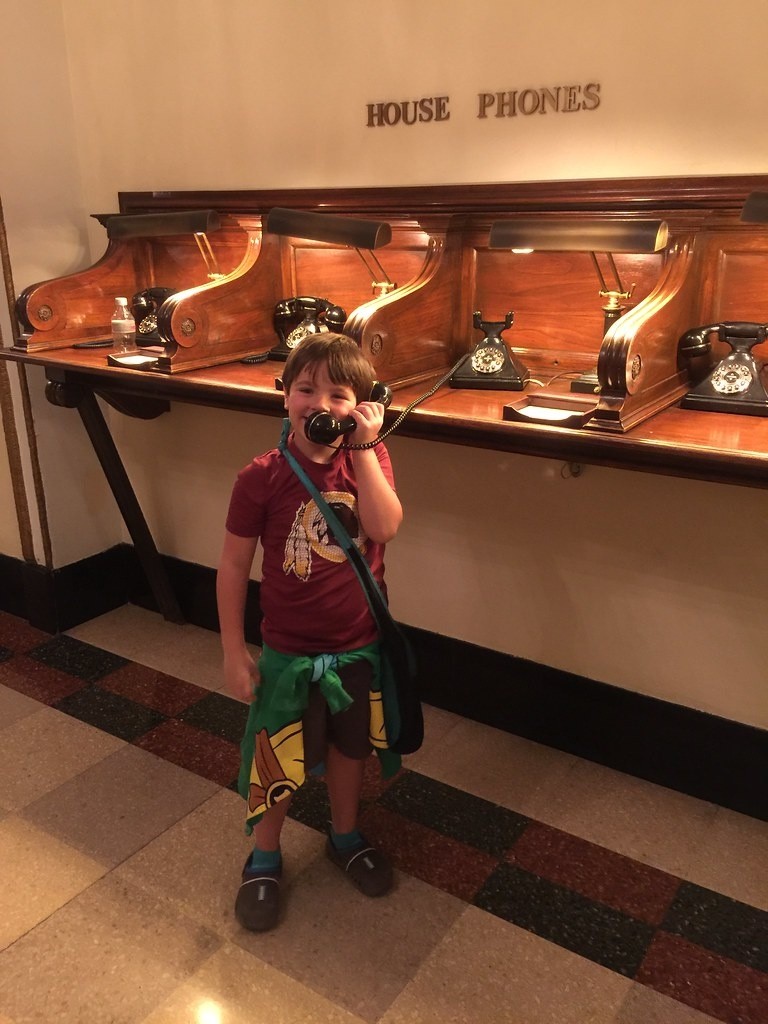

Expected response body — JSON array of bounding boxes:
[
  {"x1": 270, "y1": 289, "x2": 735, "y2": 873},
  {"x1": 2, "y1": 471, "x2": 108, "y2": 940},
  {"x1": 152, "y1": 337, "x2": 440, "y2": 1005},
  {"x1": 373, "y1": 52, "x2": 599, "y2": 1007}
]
[
  {"x1": 680, "y1": 320, "x2": 768, "y2": 417},
  {"x1": 305, "y1": 381, "x2": 394, "y2": 444},
  {"x1": 268, "y1": 296, "x2": 346, "y2": 363},
  {"x1": 132, "y1": 286, "x2": 180, "y2": 348}
]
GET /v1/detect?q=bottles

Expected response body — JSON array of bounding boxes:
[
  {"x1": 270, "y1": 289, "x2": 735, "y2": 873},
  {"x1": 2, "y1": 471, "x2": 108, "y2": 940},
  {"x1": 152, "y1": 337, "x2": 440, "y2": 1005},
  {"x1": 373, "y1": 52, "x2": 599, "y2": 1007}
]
[{"x1": 110, "y1": 296, "x2": 138, "y2": 355}]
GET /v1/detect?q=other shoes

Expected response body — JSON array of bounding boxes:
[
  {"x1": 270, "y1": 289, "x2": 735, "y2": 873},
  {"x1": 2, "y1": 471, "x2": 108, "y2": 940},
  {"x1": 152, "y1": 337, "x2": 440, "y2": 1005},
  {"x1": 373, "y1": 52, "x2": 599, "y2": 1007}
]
[
  {"x1": 324, "y1": 829, "x2": 393, "y2": 898},
  {"x1": 233, "y1": 852, "x2": 283, "y2": 931}
]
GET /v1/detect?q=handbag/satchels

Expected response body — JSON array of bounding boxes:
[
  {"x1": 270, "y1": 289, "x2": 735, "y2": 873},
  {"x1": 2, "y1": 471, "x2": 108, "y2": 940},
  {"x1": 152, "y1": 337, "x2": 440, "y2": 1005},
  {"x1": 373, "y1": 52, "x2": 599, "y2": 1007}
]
[{"x1": 377, "y1": 631, "x2": 424, "y2": 755}]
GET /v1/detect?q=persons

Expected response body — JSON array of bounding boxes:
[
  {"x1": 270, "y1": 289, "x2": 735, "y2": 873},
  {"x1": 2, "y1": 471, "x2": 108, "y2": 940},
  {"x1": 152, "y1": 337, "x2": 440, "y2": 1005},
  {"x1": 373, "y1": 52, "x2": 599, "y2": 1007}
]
[{"x1": 216, "y1": 332, "x2": 402, "y2": 934}]
